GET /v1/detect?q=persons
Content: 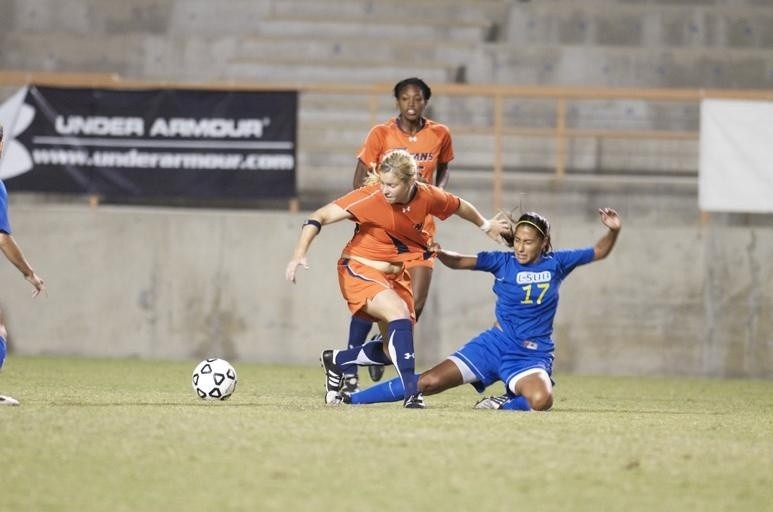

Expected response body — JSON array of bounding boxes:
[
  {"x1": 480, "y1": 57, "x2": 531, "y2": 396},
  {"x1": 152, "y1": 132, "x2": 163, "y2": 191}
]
[
  {"x1": 285, "y1": 149, "x2": 510, "y2": 408},
  {"x1": 331, "y1": 206, "x2": 622, "y2": 408},
  {"x1": 343, "y1": 77, "x2": 456, "y2": 389},
  {"x1": 0, "y1": 128, "x2": 48, "y2": 403}
]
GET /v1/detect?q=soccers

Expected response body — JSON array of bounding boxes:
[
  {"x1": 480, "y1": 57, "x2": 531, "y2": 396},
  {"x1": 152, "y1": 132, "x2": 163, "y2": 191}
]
[{"x1": 193, "y1": 359, "x2": 238, "y2": 401}]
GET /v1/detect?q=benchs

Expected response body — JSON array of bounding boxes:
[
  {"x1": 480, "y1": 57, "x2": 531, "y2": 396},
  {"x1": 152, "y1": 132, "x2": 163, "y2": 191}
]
[{"x1": 208, "y1": 1, "x2": 771, "y2": 219}]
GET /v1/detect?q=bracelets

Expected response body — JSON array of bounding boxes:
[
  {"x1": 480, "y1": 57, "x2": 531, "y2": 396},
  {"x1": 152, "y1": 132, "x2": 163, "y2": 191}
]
[{"x1": 479, "y1": 216, "x2": 490, "y2": 235}]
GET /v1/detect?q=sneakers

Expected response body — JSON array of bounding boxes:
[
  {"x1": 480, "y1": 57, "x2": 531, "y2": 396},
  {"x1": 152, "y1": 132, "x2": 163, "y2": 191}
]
[
  {"x1": 402, "y1": 392, "x2": 426, "y2": 407},
  {"x1": 344, "y1": 361, "x2": 388, "y2": 410},
  {"x1": 472, "y1": 392, "x2": 512, "y2": 411},
  {"x1": 320, "y1": 349, "x2": 342, "y2": 403}
]
